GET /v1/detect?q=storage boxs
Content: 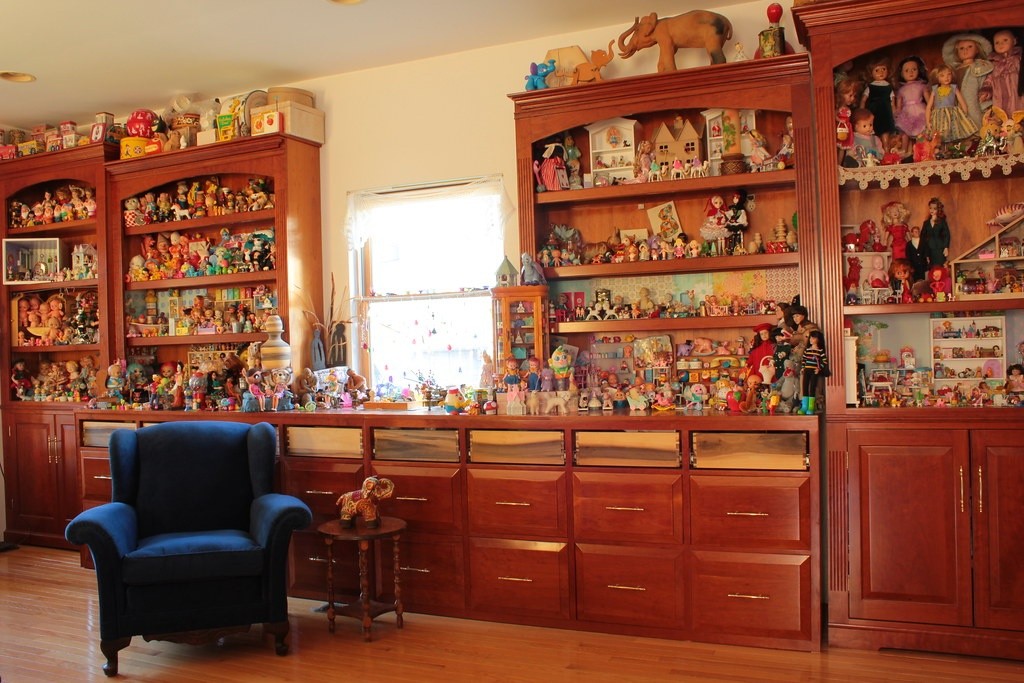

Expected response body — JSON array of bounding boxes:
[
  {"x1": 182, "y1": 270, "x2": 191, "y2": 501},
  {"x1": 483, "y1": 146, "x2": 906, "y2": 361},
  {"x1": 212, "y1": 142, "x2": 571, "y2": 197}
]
[
  {"x1": 249, "y1": 101, "x2": 325, "y2": 146},
  {"x1": 197, "y1": 128, "x2": 219, "y2": 146},
  {"x1": 3, "y1": 238, "x2": 68, "y2": 285}
]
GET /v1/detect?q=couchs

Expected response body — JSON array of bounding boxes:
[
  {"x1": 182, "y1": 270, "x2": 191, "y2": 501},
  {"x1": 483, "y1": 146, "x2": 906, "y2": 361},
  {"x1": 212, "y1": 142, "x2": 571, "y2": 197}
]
[{"x1": 64, "y1": 423, "x2": 313, "y2": 676}]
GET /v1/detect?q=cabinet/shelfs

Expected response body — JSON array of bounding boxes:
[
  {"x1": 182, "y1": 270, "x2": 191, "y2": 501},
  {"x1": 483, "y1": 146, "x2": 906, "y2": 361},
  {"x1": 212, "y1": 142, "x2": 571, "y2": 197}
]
[{"x1": 0, "y1": 0, "x2": 1024, "y2": 660}]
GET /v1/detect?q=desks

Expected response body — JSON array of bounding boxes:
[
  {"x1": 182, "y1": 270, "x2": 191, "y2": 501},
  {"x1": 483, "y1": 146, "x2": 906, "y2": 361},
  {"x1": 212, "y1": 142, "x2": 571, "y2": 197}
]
[{"x1": 319, "y1": 515, "x2": 406, "y2": 641}]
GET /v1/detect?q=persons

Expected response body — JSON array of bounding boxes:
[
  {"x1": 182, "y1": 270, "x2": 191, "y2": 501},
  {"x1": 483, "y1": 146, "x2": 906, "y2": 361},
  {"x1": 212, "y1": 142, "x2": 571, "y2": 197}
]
[
  {"x1": 745, "y1": 306, "x2": 828, "y2": 415},
  {"x1": 504, "y1": 345, "x2": 735, "y2": 413},
  {"x1": 540, "y1": 189, "x2": 750, "y2": 266},
  {"x1": 11, "y1": 356, "x2": 369, "y2": 411},
  {"x1": 868, "y1": 198, "x2": 952, "y2": 304},
  {"x1": 476, "y1": 351, "x2": 494, "y2": 392},
  {"x1": 547, "y1": 288, "x2": 780, "y2": 325},
  {"x1": 140, "y1": 192, "x2": 171, "y2": 224},
  {"x1": 17, "y1": 297, "x2": 68, "y2": 347},
  {"x1": 864, "y1": 341, "x2": 1024, "y2": 406},
  {"x1": 833, "y1": 30, "x2": 1024, "y2": 168},
  {"x1": 562, "y1": 115, "x2": 805, "y2": 190},
  {"x1": 127, "y1": 232, "x2": 275, "y2": 284},
  {"x1": 9, "y1": 185, "x2": 97, "y2": 229},
  {"x1": 190, "y1": 295, "x2": 257, "y2": 336}
]
